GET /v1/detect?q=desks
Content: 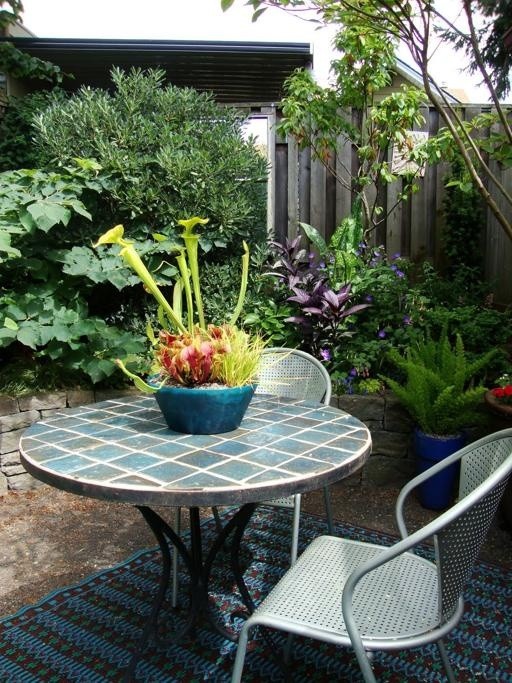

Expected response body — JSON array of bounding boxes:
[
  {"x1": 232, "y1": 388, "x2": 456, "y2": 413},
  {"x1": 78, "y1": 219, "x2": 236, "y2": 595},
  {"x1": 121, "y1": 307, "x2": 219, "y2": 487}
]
[{"x1": 19, "y1": 395, "x2": 374, "y2": 683}]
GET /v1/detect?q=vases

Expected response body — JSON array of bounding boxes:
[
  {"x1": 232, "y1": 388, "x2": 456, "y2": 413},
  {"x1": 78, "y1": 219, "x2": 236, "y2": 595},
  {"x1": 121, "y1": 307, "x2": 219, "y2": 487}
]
[
  {"x1": 485, "y1": 390, "x2": 512, "y2": 430},
  {"x1": 141, "y1": 375, "x2": 259, "y2": 434}
]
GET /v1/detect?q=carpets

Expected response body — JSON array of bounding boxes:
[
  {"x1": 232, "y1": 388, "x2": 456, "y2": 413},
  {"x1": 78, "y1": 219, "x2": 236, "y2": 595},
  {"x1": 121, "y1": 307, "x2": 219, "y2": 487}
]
[{"x1": 0, "y1": 495, "x2": 512, "y2": 683}]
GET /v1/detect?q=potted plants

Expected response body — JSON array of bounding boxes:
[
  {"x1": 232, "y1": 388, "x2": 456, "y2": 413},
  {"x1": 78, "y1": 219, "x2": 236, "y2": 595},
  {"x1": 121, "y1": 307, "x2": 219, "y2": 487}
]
[{"x1": 375, "y1": 314, "x2": 501, "y2": 512}]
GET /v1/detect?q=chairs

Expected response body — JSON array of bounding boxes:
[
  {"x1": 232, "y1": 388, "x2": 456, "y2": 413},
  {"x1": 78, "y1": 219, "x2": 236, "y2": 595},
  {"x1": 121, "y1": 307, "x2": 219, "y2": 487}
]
[
  {"x1": 173, "y1": 346, "x2": 334, "y2": 567},
  {"x1": 231, "y1": 426, "x2": 510, "y2": 683}
]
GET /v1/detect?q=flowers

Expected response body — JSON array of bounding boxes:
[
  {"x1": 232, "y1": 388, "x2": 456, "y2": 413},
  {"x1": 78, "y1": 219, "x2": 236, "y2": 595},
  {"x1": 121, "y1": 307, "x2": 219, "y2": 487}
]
[
  {"x1": 88, "y1": 213, "x2": 314, "y2": 394},
  {"x1": 491, "y1": 373, "x2": 512, "y2": 406}
]
